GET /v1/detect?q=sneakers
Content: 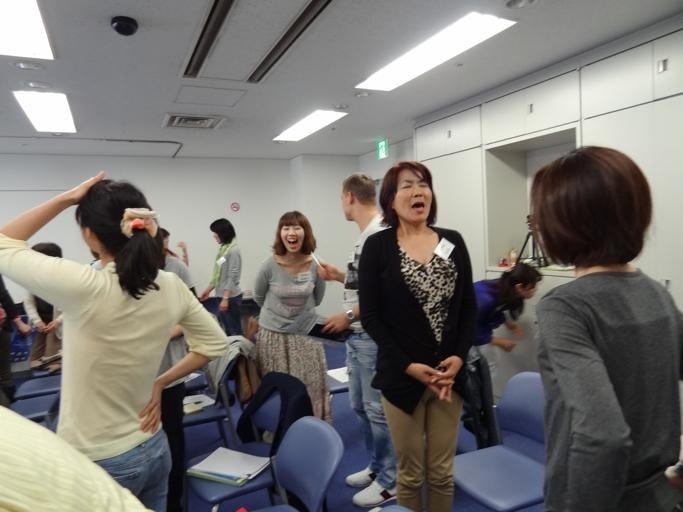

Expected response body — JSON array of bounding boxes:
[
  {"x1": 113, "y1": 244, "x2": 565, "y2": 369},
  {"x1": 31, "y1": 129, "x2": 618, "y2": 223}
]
[
  {"x1": 344, "y1": 465, "x2": 382, "y2": 489},
  {"x1": 351, "y1": 475, "x2": 400, "y2": 508}
]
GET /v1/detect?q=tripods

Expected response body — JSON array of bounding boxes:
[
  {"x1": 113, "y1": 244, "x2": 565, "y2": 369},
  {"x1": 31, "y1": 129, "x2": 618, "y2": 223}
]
[{"x1": 514, "y1": 231, "x2": 549, "y2": 269}]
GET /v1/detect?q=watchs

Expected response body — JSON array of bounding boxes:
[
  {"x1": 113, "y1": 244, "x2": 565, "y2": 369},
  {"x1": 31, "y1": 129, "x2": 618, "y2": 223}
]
[{"x1": 344, "y1": 307, "x2": 356, "y2": 323}]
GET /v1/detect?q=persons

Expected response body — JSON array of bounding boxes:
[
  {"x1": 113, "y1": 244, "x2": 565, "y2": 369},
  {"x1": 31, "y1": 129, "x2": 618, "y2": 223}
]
[
  {"x1": 21, "y1": 242, "x2": 63, "y2": 361},
  {"x1": 467, "y1": 262, "x2": 541, "y2": 449},
  {"x1": 90, "y1": 250, "x2": 196, "y2": 511},
  {"x1": 251, "y1": 210, "x2": 331, "y2": 426},
  {"x1": 157, "y1": 227, "x2": 189, "y2": 265},
  {"x1": 1, "y1": 171, "x2": 227, "y2": 511},
  {"x1": 317, "y1": 172, "x2": 400, "y2": 505},
  {"x1": 0, "y1": 270, "x2": 30, "y2": 408},
  {"x1": 358, "y1": 159, "x2": 480, "y2": 511},
  {"x1": 525, "y1": 144, "x2": 681, "y2": 511},
  {"x1": 199, "y1": 219, "x2": 246, "y2": 335}
]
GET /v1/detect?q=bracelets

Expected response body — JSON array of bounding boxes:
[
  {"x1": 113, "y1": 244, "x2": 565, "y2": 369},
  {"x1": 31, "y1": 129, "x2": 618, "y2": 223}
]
[{"x1": 155, "y1": 377, "x2": 166, "y2": 390}]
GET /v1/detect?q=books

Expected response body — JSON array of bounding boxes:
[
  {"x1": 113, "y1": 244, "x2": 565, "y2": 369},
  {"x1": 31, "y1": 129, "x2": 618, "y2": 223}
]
[
  {"x1": 189, "y1": 444, "x2": 271, "y2": 480},
  {"x1": 181, "y1": 403, "x2": 203, "y2": 415},
  {"x1": 184, "y1": 467, "x2": 250, "y2": 489},
  {"x1": 182, "y1": 393, "x2": 216, "y2": 408},
  {"x1": 326, "y1": 365, "x2": 349, "y2": 383}
]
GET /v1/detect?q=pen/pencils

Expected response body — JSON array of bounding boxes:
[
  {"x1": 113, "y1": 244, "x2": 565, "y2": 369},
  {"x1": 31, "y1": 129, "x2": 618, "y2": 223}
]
[{"x1": 311, "y1": 252, "x2": 320, "y2": 266}]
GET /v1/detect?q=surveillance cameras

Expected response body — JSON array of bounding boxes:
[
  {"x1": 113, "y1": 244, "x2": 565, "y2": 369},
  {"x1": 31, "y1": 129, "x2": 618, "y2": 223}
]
[{"x1": 110, "y1": 15, "x2": 137, "y2": 37}]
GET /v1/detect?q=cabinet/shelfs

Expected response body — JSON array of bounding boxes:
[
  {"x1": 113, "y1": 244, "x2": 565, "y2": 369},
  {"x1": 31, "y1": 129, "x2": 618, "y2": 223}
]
[
  {"x1": 481, "y1": 259, "x2": 577, "y2": 400},
  {"x1": 480, "y1": 63, "x2": 581, "y2": 151},
  {"x1": 411, "y1": 101, "x2": 533, "y2": 292},
  {"x1": 577, "y1": 23, "x2": 682, "y2": 321}
]
